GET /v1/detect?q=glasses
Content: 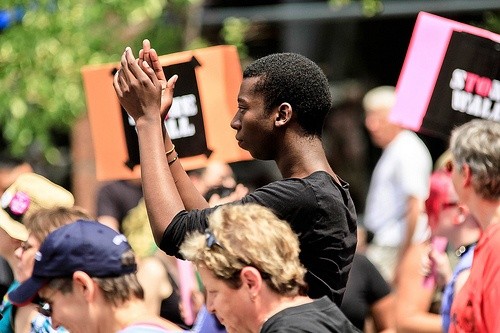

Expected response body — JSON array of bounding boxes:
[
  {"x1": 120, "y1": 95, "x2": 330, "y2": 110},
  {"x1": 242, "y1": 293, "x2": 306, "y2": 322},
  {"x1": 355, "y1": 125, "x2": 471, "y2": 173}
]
[
  {"x1": 37, "y1": 282, "x2": 68, "y2": 317},
  {"x1": 204, "y1": 228, "x2": 225, "y2": 251},
  {"x1": 446, "y1": 160, "x2": 462, "y2": 172}
]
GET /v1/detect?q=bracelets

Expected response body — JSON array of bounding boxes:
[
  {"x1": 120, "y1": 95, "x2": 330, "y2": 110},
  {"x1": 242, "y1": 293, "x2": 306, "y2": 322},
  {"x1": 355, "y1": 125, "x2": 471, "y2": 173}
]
[
  {"x1": 165, "y1": 144, "x2": 175, "y2": 156},
  {"x1": 168, "y1": 152, "x2": 178, "y2": 166}
]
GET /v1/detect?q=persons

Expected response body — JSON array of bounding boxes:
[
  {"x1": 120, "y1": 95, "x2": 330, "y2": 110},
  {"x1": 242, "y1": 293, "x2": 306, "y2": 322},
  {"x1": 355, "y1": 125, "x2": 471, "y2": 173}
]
[{"x1": 0, "y1": 39, "x2": 500, "y2": 333}]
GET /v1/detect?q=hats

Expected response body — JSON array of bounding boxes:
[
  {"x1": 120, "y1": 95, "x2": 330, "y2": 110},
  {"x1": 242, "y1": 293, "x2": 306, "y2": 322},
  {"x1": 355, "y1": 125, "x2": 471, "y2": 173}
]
[
  {"x1": 0, "y1": 174, "x2": 77, "y2": 242},
  {"x1": 7, "y1": 218, "x2": 138, "y2": 306}
]
